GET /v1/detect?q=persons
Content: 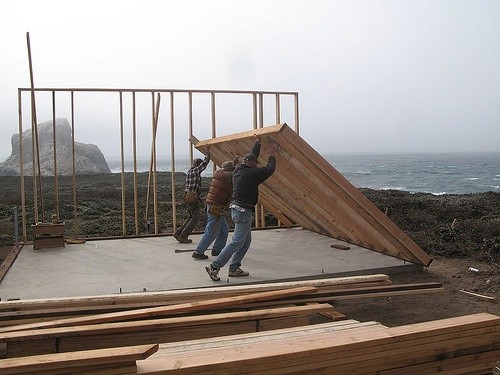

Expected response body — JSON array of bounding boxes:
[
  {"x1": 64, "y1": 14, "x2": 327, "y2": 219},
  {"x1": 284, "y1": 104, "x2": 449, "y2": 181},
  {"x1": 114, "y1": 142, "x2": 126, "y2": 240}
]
[
  {"x1": 192, "y1": 153, "x2": 241, "y2": 259},
  {"x1": 205, "y1": 138, "x2": 278, "y2": 281},
  {"x1": 173, "y1": 147, "x2": 211, "y2": 243}
]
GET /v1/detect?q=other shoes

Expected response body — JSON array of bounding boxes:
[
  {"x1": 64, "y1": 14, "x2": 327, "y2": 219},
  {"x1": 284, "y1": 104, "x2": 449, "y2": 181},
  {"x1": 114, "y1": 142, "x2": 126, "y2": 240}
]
[
  {"x1": 178, "y1": 237, "x2": 192, "y2": 243},
  {"x1": 174, "y1": 233, "x2": 180, "y2": 241},
  {"x1": 192, "y1": 252, "x2": 208, "y2": 259},
  {"x1": 212, "y1": 249, "x2": 220, "y2": 256}
]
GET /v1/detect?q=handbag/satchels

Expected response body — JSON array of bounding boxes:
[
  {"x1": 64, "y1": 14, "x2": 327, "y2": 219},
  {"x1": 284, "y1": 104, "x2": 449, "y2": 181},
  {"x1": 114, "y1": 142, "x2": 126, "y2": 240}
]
[
  {"x1": 209, "y1": 204, "x2": 225, "y2": 216},
  {"x1": 183, "y1": 192, "x2": 197, "y2": 203}
]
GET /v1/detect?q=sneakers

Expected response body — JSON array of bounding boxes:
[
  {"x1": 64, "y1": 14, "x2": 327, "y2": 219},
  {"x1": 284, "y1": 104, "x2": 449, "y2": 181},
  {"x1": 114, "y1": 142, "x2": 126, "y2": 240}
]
[
  {"x1": 228, "y1": 267, "x2": 249, "y2": 277},
  {"x1": 205, "y1": 264, "x2": 220, "y2": 280}
]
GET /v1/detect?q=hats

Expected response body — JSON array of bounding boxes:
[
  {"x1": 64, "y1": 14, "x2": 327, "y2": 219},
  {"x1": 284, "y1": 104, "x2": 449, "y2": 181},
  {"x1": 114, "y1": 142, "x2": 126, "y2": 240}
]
[{"x1": 243, "y1": 153, "x2": 261, "y2": 164}]
